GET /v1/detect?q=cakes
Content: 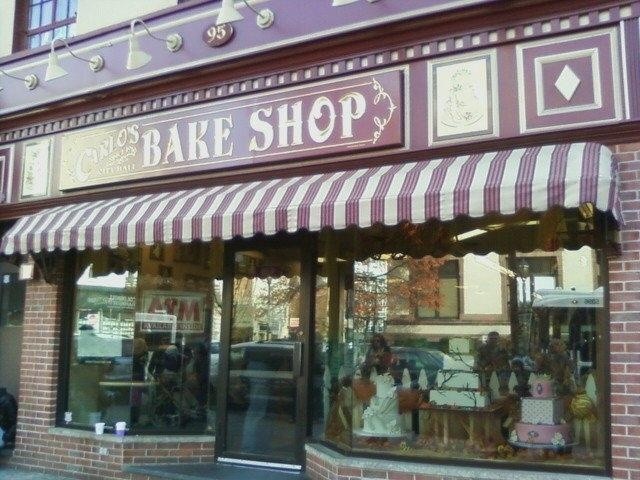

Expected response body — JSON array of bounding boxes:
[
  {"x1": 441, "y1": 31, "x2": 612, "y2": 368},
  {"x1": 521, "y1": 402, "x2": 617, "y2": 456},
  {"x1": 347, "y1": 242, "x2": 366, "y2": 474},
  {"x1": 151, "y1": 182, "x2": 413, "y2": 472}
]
[
  {"x1": 362, "y1": 373, "x2": 402, "y2": 435},
  {"x1": 429, "y1": 337, "x2": 490, "y2": 408},
  {"x1": 515, "y1": 372, "x2": 571, "y2": 445}
]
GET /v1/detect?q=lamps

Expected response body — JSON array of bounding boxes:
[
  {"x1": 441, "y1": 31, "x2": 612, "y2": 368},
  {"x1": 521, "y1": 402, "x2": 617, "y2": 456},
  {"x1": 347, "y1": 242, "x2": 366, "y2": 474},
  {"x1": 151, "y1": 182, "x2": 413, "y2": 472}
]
[
  {"x1": 124, "y1": 18, "x2": 184, "y2": 70},
  {"x1": 329, "y1": 0, "x2": 379, "y2": 9},
  {"x1": 0, "y1": 68, "x2": 39, "y2": 91},
  {"x1": 42, "y1": 35, "x2": 108, "y2": 82},
  {"x1": 216, "y1": 0, "x2": 275, "y2": 31}
]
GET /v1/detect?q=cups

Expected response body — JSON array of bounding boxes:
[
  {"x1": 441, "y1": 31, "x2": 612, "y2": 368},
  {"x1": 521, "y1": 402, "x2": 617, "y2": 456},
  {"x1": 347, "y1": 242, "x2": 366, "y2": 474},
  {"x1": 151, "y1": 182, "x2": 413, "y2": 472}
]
[
  {"x1": 115, "y1": 422, "x2": 128, "y2": 437},
  {"x1": 95, "y1": 422, "x2": 105, "y2": 434}
]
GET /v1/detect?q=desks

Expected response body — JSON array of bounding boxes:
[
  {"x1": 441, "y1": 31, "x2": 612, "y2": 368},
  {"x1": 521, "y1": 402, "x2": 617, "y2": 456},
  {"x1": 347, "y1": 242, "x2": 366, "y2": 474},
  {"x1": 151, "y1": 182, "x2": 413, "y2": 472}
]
[
  {"x1": 417, "y1": 401, "x2": 506, "y2": 445},
  {"x1": 98, "y1": 379, "x2": 162, "y2": 422}
]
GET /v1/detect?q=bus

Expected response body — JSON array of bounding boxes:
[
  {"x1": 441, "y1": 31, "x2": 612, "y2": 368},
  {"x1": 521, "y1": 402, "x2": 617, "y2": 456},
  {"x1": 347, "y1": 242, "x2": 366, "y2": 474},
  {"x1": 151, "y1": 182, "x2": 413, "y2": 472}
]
[{"x1": 529, "y1": 287, "x2": 605, "y2": 378}]
